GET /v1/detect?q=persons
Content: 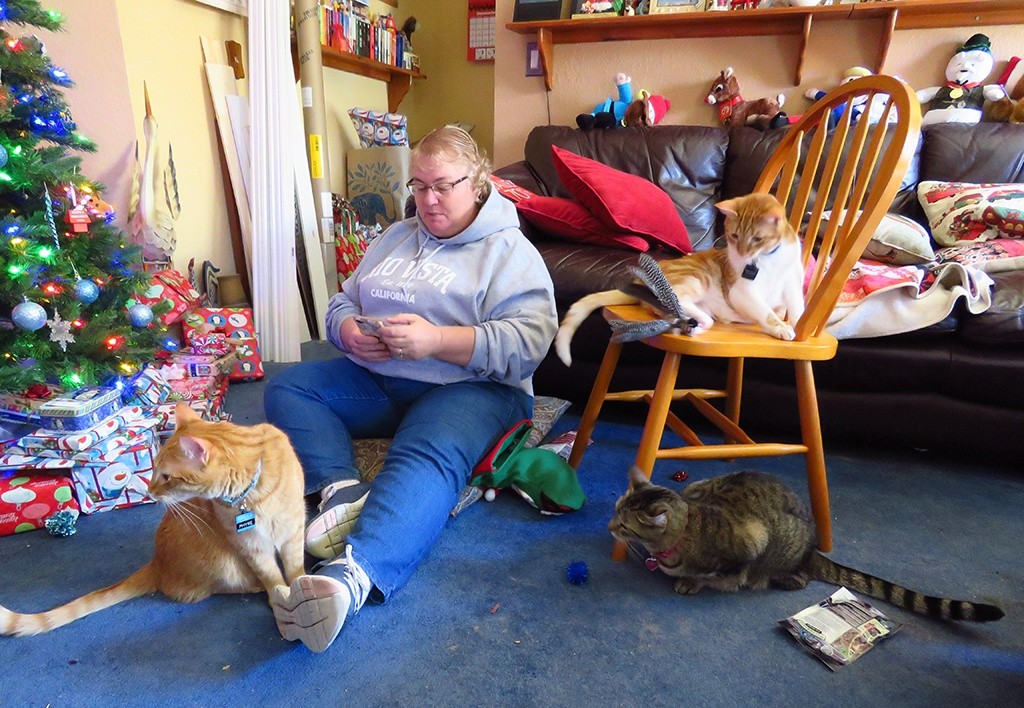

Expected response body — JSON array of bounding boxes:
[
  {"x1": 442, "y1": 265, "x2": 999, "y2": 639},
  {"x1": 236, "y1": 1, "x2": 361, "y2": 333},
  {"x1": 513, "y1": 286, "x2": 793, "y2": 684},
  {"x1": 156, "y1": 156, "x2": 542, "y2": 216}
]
[{"x1": 260, "y1": 126, "x2": 560, "y2": 656}]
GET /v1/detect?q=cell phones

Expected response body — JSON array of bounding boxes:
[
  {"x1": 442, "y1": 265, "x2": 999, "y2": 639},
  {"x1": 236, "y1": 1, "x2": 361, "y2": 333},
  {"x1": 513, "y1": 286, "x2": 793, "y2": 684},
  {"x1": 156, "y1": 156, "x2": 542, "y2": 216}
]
[{"x1": 353, "y1": 314, "x2": 384, "y2": 342}]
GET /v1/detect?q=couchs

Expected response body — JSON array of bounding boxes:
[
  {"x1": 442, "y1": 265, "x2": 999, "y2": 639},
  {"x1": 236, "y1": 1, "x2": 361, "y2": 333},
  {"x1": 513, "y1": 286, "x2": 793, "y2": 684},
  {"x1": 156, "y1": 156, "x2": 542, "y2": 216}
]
[{"x1": 483, "y1": 121, "x2": 1024, "y2": 467}]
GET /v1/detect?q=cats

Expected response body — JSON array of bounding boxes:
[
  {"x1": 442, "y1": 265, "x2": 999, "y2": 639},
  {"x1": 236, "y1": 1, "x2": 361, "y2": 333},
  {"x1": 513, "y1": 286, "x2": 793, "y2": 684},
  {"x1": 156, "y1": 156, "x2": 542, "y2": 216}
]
[
  {"x1": 606, "y1": 465, "x2": 1004, "y2": 635},
  {"x1": 1, "y1": 399, "x2": 307, "y2": 651},
  {"x1": 555, "y1": 190, "x2": 808, "y2": 367}
]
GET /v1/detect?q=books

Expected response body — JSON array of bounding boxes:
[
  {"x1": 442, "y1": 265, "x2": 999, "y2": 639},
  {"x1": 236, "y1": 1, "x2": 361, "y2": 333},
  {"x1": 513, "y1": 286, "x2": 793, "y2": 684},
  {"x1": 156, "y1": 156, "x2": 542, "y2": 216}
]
[{"x1": 318, "y1": 0, "x2": 426, "y2": 74}]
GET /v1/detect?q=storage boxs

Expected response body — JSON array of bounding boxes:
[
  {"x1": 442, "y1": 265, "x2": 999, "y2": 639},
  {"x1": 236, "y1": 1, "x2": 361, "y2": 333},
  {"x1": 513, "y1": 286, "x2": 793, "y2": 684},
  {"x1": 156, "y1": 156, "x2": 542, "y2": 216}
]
[
  {"x1": 1, "y1": 307, "x2": 265, "y2": 537},
  {"x1": 347, "y1": 107, "x2": 410, "y2": 232}
]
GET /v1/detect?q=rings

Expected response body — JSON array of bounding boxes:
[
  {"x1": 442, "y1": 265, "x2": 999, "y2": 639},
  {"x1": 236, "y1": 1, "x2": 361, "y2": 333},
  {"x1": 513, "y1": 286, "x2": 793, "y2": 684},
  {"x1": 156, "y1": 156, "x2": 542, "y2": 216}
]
[{"x1": 397, "y1": 348, "x2": 405, "y2": 359}]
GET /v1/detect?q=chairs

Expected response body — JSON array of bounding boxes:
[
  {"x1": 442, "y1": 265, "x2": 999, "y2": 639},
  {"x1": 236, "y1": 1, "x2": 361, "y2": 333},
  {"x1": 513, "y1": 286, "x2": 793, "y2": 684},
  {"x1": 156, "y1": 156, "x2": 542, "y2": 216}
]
[{"x1": 565, "y1": 70, "x2": 922, "y2": 565}]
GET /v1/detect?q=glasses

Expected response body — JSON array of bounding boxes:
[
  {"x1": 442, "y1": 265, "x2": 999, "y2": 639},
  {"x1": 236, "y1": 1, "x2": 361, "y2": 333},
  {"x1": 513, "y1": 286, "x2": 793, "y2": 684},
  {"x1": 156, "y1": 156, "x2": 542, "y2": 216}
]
[{"x1": 406, "y1": 176, "x2": 468, "y2": 197}]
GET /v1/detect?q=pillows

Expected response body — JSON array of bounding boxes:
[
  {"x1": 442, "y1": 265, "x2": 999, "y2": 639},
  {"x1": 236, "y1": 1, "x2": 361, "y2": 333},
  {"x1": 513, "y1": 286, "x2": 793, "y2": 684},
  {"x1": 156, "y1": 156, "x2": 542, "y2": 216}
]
[
  {"x1": 805, "y1": 209, "x2": 935, "y2": 266},
  {"x1": 930, "y1": 238, "x2": 1024, "y2": 275},
  {"x1": 514, "y1": 196, "x2": 651, "y2": 253},
  {"x1": 916, "y1": 178, "x2": 1024, "y2": 248},
  {"x1": 549, "y1": 144, "x2": 694, "y2": 257}
]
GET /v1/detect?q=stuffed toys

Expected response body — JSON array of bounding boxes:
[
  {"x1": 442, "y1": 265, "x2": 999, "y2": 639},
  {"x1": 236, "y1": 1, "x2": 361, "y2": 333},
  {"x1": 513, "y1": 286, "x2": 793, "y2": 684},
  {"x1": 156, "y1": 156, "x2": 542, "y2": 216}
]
[
  {"x1": 802, "y1": 65, "x2": 876, "y2": 130},
  {"x1": 578, "y1": 72, "x2": 671, "y2": 129},
  {"x1": 917, "y1": 33, "x2": 996, "y2": 128},
  {"x1": 705, "y1": 66, "x2": 786, "y2": 128}
]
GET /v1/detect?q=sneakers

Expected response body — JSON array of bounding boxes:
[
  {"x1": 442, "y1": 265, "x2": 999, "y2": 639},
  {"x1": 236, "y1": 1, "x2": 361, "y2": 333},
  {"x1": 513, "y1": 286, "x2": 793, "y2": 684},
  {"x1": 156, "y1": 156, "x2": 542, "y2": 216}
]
[
  {"x1": 304, "y1": 481, "x2": 373, "y2": 561},
  {"x1": 271, "y1": 543, "x2": 371, "y2": 655}
]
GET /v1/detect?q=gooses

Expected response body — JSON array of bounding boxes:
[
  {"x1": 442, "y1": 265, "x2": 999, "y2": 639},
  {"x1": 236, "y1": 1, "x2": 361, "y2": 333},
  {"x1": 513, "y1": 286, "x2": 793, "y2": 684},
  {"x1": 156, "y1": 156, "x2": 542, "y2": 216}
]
[{"x1": 128, "y1": 77, "x2": 181, "y2": 267}]
